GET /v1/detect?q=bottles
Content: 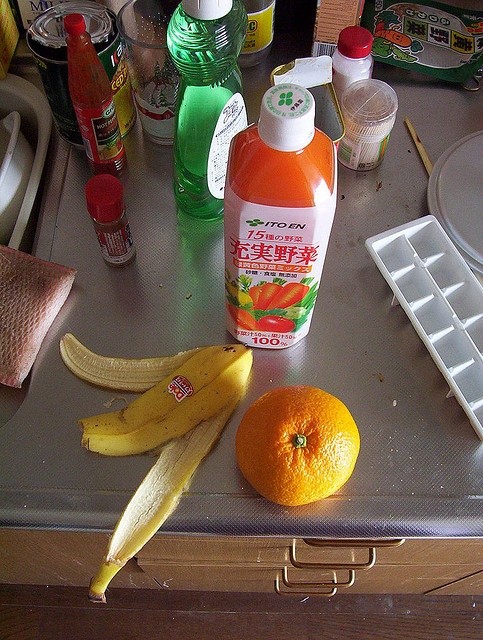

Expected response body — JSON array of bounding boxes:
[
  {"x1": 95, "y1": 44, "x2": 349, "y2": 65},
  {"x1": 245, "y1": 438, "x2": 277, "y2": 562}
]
[
  {"x1": 337, "y1": 78, "x2": 399, "y2": 172},
  {"x1": 235, "y1": 0, "x2": 276, "y2": 69},
  {"x1": 84, "y1": 172, "x2": 137, "y2": 269},
  {"x1": 331, "y1": 25, "x2": 375, "y2": 102},
  {"x1": 63, "y1": 13, "x2": 128, "y2": 181},
  {"x1": 223, "y1": 82, "x2": 339, "y2": 349},
  {"x1": 165, "y1": 0, "x2": 249, "y2": 219}
]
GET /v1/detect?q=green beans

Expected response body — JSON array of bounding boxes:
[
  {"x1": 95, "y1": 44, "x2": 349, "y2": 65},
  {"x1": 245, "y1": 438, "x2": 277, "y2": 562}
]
[{"x1": 393, "y1": 46, "x2": 417, "y2": 62}]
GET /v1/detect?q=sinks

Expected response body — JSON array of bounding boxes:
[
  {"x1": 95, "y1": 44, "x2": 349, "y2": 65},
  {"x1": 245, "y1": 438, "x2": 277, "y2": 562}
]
[{"x1": 0, "y1": 56, "x2": 71, "y2": 263}]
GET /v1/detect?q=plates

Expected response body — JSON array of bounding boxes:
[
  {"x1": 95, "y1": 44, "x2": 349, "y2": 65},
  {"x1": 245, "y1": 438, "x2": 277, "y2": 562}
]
[
  {"x1": 436, "y1": 132, "x2": 483, "y2": 266},
  {"x1": 426, "y1": 130, "x2": 483, "y2": 274}
]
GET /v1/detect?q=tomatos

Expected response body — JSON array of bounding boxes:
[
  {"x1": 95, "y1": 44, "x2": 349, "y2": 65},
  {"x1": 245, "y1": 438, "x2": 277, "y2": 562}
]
[{"x1": 256, "y1": 316, "x2": 294, "y2": 332}]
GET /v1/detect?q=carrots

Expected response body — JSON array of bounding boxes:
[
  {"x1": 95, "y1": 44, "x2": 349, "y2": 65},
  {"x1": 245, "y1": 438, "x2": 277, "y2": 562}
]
[
  {"x1": 374, "y1": 30, "x2": 412, "y2": 47},
  {"x1": 257, "y1": 283, "x2": 281, "y2": 310},
  {"x1": 251, "y1": 287, "x2": 262, "y2": 307},
  {"x1": 266, "y1": 283, "x2": 309, "y2": 309}
]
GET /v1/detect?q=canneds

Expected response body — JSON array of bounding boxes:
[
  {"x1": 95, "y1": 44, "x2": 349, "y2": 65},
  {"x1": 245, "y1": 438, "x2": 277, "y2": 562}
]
[
  {"x1": 27, "y1": 2, "x2": 136, "y2": 148},
  {"x1": 237, "y1": 0, "x2": 274, "y2": 57}
]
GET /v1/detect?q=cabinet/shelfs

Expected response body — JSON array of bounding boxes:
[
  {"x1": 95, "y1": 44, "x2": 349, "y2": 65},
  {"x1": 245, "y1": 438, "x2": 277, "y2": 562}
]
[{"x1": 134, "y1": 537, "x2": 483, "y2": 597}]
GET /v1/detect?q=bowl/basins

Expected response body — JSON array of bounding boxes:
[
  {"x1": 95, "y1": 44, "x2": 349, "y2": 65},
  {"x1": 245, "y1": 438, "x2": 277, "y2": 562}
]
[{"x1": 0, "y1": 110, "x2": 34, "y2": 247}]
